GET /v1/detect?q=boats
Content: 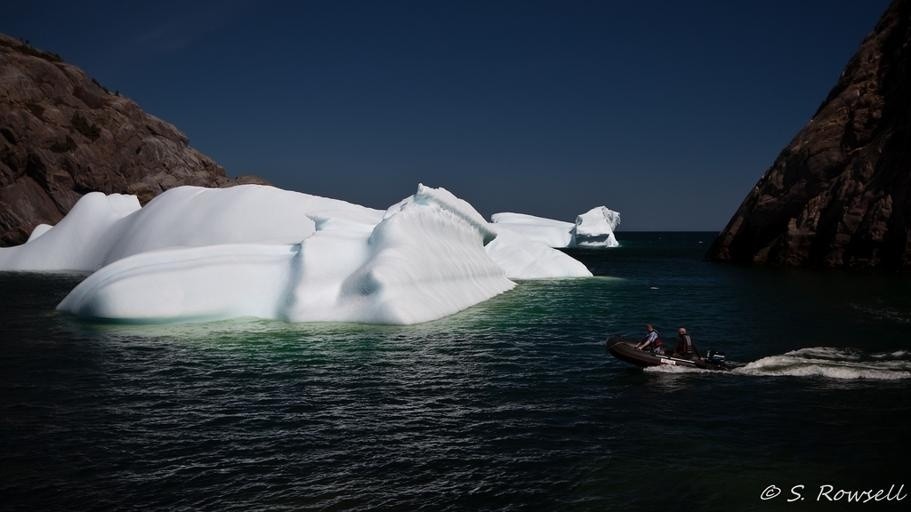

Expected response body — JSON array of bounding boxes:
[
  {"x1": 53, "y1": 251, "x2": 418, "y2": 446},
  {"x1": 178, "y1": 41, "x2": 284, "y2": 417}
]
[{"x1": 606, "y1": 336, "x2": 731, "y2": 373}]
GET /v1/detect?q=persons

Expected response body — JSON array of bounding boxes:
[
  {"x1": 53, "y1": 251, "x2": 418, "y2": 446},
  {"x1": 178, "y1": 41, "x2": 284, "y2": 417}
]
[
  {"x1": 670, "y1": 326, "x2": 707, "y2": 365},
  {"x1": 634, "y1": 322, "x2": 667, "y2": 357}
]
